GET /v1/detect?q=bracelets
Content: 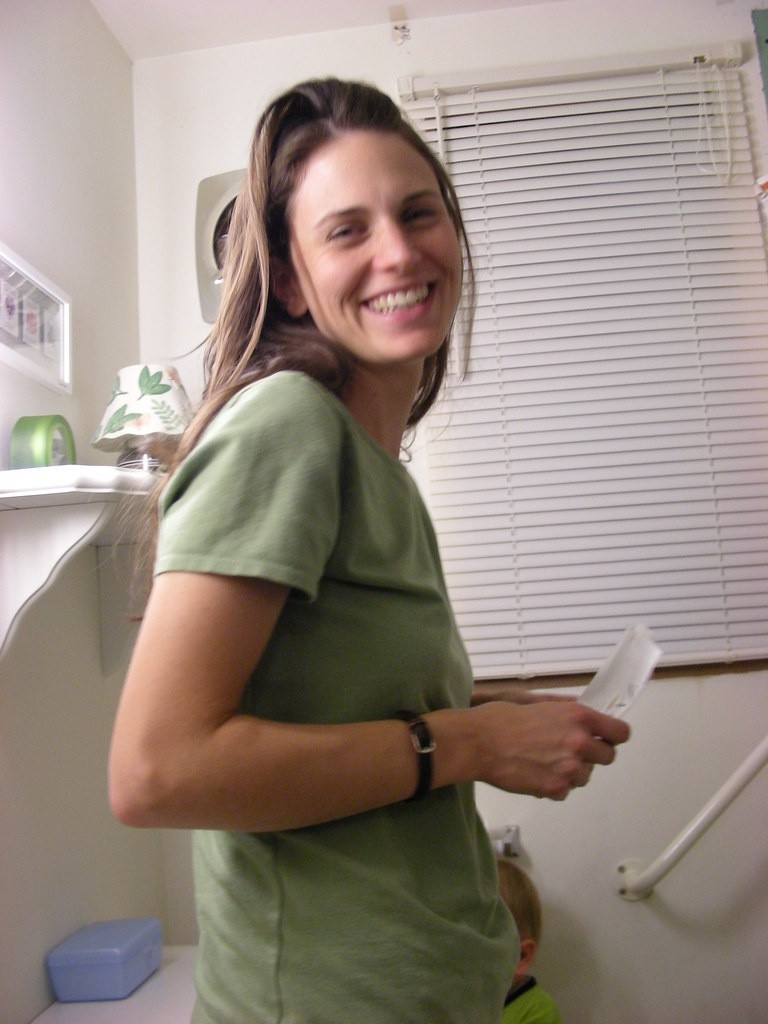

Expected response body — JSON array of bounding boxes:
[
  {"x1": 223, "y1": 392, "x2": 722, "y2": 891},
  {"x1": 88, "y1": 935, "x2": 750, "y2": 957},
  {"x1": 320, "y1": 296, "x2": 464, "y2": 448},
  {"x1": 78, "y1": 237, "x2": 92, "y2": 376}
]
[{"x1": 391, "y1": 710, "x2": 437, "y2": 802}]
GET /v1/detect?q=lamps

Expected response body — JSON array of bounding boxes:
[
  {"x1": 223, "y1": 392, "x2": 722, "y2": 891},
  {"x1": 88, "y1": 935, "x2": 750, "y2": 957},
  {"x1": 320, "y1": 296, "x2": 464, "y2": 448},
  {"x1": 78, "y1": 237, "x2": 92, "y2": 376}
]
[{"x1": 91, "y1": 364, "x2": 197, "y2": 471}]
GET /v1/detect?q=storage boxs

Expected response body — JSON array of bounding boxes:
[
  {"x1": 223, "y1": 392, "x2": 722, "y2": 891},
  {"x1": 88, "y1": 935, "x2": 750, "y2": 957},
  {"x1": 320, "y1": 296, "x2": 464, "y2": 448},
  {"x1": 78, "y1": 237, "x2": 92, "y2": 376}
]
[{"x1": 50, "y1": 918, "x2": 164, "y2": 1003}]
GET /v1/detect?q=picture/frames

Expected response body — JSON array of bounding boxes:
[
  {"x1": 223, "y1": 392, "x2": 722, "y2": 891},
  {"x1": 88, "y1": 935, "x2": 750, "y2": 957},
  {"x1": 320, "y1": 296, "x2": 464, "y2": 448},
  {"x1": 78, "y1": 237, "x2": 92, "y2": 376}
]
[{"x1": 1, "y1": 244, "x2": 72, "y2": 397}]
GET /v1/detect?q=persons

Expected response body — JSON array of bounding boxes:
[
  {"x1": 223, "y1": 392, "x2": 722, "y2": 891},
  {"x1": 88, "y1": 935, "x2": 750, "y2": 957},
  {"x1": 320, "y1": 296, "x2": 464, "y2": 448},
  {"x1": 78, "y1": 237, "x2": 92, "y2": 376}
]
[
  {"x1": 495, "y1": 857, "x2": 563, "y2": 1024},
  {"x1": 107, "y1": 79, "x2": 629, "y2": 1024}
]
